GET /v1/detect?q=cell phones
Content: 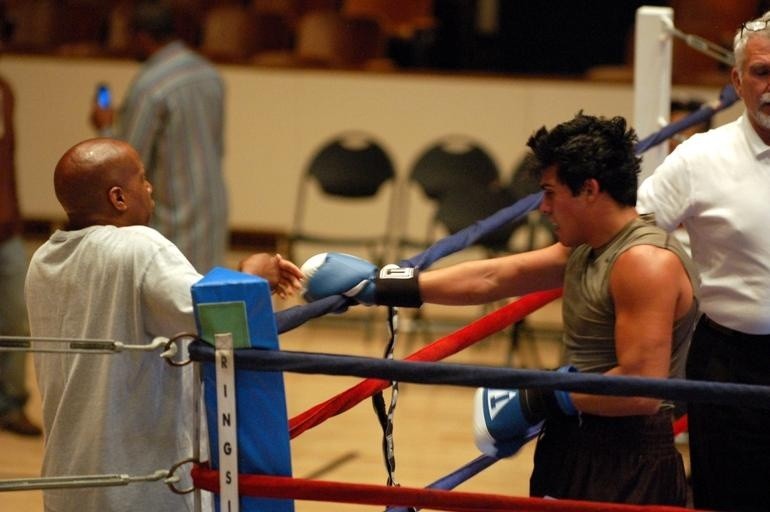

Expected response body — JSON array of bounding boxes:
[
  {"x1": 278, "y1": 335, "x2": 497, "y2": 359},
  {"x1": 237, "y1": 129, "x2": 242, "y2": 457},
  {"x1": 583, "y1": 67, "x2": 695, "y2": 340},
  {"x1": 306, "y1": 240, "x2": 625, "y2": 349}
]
[{"x1": 98, "y1": 85, "x2": 110, "y2": 109}]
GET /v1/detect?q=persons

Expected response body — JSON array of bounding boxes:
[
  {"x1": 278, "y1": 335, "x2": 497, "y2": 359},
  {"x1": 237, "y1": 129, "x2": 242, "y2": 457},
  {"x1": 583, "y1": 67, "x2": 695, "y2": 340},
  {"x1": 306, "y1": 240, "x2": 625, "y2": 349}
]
[
  {"x1": 89, "y1": 2, "x2": 230, "y2": 274},
  {"x1": 634, "y1": 1, "x2": 769, "y2": 512},
  {"x1": 300, "y1": 109, "x2": 702, "y2": 506},
  {"x1": 22, "y1": 138, "x2": 305, "y2": 511}
]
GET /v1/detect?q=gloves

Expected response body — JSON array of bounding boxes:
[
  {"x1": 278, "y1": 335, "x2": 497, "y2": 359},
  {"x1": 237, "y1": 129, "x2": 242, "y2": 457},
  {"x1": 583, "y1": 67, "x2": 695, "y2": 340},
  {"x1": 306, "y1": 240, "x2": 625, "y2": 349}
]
[
  {"x1": 298, "y1": 252, "x2": 423, "y2": 314},
  {"x1": 473, "y1": 365, "x2": 582, "y2": 459}
]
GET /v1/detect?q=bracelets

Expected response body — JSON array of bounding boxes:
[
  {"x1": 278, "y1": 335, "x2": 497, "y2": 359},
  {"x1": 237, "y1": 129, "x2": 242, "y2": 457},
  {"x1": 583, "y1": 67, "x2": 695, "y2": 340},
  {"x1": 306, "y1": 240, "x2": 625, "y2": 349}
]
[
  {"x1": 372, "y1": 265, "x2": 423, "y2": 308},
  {"x1": 516, "y1": 386, "x2": 571, "y2": 426}
]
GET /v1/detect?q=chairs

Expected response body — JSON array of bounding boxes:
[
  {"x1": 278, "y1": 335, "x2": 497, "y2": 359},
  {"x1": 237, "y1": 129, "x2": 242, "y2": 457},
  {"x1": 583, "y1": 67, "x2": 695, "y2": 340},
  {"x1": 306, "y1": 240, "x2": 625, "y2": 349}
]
[{"x1": 279, "y1": 126, "x2": 556, "y2": 355}]
